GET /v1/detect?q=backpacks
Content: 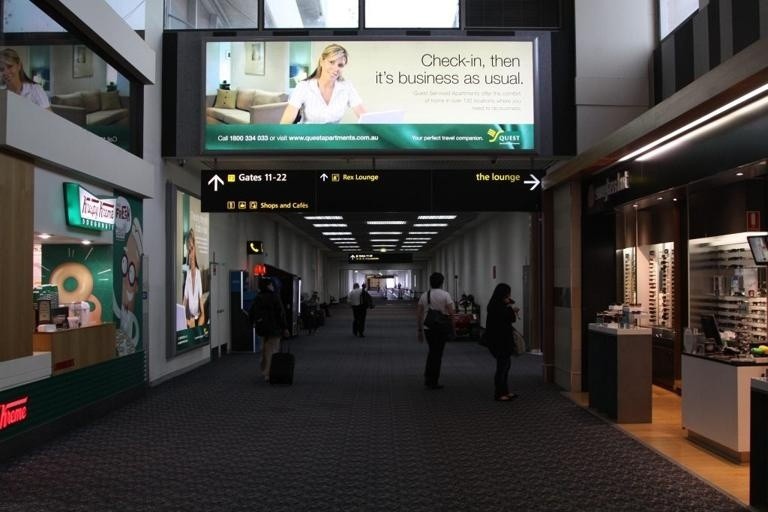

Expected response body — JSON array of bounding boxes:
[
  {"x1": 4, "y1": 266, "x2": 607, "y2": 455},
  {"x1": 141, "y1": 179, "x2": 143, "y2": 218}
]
[{"x1": 359, "y1": 289, "x2": 373, "y2": 310}]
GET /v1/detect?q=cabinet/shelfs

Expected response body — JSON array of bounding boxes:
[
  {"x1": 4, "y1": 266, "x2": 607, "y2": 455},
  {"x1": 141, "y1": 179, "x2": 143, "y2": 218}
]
[
  {"x1": 639, "y1": 248, "x2": 674, "y2": 392},
  {"x1": 716, "y1": 243, "x2": 768, "y2": 356}
]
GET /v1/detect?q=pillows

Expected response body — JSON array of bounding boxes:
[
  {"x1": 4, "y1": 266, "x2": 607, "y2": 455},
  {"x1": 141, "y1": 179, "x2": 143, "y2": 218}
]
[
  {"x1": 236, "y1": 88, "x2": 255, "y2": 112},
  {"x1": 215, "y1": 88, "x2": 239, "y2": 109},
  {"x1": 99, "y1": 90, "x2": 123, "y2": 111},
  {"x1": 82, "y1": 90, "x2": 100, "y2": 113}
]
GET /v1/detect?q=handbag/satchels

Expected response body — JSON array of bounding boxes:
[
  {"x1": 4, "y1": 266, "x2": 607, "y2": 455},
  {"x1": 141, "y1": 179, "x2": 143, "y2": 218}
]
[
  {"x1": 423, "y1": 308, "x2": 453, "y2": 337},
  {"x1": 512, "y1": 328, "x2": 526, "y2": 356}
]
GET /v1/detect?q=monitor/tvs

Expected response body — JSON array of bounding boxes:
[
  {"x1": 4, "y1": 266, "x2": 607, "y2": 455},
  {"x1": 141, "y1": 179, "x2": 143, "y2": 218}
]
[
  {"x1": 699, "y1": 312, "x2": 722, "y2": 346},
  {"x1": 747, "y1": 234, "x2": 768, "y2": 265}
]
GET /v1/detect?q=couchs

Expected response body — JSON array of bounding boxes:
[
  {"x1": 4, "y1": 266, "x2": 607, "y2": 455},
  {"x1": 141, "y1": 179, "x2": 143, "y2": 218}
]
[
  {"x1": 205, "y1": 92, "x2": 289, "y2": 124},
  {"x1": 50, "y1": 95, "x2": 132, "y2": 137}
]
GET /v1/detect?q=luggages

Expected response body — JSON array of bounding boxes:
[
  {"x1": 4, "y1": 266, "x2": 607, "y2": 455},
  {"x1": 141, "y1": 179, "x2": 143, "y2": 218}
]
[{"x1": 268, "y1": 336, "x2": 295, "y2": 386}]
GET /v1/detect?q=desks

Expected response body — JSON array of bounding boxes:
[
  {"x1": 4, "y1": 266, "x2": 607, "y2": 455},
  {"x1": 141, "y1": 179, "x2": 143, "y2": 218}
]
[
  {"x1": 750, "y1": 377, "x2": 768, "y2": 510},
  {"x1": 33, "y1": 320, "x2": 117, "y2": 373},
  {"x1": 681, "y1": 350, "x2": 768, "y2": 466},
  {"x1": 587, "y1": 322, "x2": 652, "y2": 423}
]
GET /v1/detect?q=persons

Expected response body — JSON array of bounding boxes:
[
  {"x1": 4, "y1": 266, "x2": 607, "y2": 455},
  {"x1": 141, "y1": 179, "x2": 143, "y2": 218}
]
[
  {"x1": 183, "y1": 227, "x2": 210, "y2": 327},
  {"x1": 247, "y1": 275, "x2": 290, "y2": 384},
  {"x1": 0, "y1": 48, "x2": 54, "y2": 112},
  {"x1": 112, "y1": 217, "x2": 144, "y2": 357},
  {"x1": 416, "y1": 271, "x2": 456, "y2": 389},
  {"x1": 348, "y1": 283, "x2": 365, "y2": 337},
  {"x1": 478, "y1": 283, "x2": 520, "y2": 402},
  {"x1": 307, "y1": 291, "x2": 320, "y2": 336},
  {"x1": 280, "y1": 45, "x2": 371, "y2": 124}
]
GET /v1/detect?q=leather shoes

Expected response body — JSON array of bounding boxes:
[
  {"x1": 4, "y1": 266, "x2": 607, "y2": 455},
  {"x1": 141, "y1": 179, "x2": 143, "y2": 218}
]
[
  {"x1": 424, "y1": 382, "x2": 445, "y2": 391},
  {"x1": 506, "y1": 392, "x2": 518, "y2": 398},
  {"x1": 493, "y1": 396, "x2": 515, "y2": 402}
]
